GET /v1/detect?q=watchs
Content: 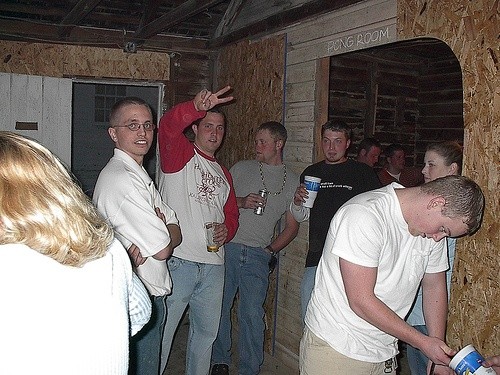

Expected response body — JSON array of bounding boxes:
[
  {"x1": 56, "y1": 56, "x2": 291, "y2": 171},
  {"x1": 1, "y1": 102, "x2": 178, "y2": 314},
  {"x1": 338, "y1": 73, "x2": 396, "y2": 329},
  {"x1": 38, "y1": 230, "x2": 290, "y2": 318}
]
[{"x1": 266, "y1": 245, "x2": 277, "y2": 257}]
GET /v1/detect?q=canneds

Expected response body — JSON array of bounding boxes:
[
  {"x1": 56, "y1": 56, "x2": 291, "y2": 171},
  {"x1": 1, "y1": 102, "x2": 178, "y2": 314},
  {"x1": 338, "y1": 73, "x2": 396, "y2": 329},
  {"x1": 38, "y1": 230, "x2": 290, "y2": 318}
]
[{"x1": 254, "y1": 189, "x2": 268, "y2": 215}]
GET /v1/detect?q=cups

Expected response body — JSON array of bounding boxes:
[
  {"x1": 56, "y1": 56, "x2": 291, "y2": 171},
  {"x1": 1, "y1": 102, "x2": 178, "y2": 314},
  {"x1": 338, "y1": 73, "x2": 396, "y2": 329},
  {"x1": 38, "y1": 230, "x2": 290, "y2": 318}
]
[
  {"x1": 204, "y1": 222, "x2": 221, "y2": 252},
  {"x1": 449, "y1": 344, "x2": 496, "y2": 375},
  {"x1": 301, "y1": 175, "x2": 322, "y2": 208}
]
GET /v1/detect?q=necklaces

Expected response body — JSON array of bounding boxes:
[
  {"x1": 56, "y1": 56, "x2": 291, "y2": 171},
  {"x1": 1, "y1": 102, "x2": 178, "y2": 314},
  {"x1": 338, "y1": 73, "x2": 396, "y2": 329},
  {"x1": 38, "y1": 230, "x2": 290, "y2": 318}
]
[{"x1": 259, "y1": 161, "x2": 287, "y2": 195}]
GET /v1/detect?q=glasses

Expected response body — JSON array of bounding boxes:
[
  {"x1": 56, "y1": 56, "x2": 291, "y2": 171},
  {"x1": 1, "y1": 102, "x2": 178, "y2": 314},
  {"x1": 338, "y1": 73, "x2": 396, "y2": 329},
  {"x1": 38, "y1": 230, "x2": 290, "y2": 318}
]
[{"x1": 113, "y1": 123, "x2": 155, "y2": 131}]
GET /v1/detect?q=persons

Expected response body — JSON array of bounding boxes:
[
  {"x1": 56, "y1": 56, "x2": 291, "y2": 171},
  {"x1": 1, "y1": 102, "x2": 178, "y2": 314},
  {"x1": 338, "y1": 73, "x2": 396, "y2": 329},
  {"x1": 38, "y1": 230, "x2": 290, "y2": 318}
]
[
  {"x1": 356, "y1": 137, "x2": 381, "y2": 167},
  {"x1": 92, "y1": 96, "x2": 183, "y2": 375},
  {"x1": 0, "y1": 131, "x2": 152, "y2": 375},
  {"x1": 158, "y1": 86, "x2": 240, "y2": 375},
  {"x1": 299, "y1": 175, "x2": 485, "y2": 375},
  {"x1": 290, "y1": 119, "x2": 383, "y2": 335},
  {"x1": 376, "y1": 143, "x2": 419, "y2": 188},
  {"x1": 405, "y1": 141, "x2": 464, "y2": 375},
  {"x1": 211, "y1": 121, "x2": 301, "y2": 375}
]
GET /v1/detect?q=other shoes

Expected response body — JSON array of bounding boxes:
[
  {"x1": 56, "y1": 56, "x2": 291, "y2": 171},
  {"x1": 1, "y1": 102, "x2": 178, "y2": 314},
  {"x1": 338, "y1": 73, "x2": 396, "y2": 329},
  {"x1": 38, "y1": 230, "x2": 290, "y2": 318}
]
[{"x1": 212, "y1": 364, "x2": 229, "y2": 375}]
